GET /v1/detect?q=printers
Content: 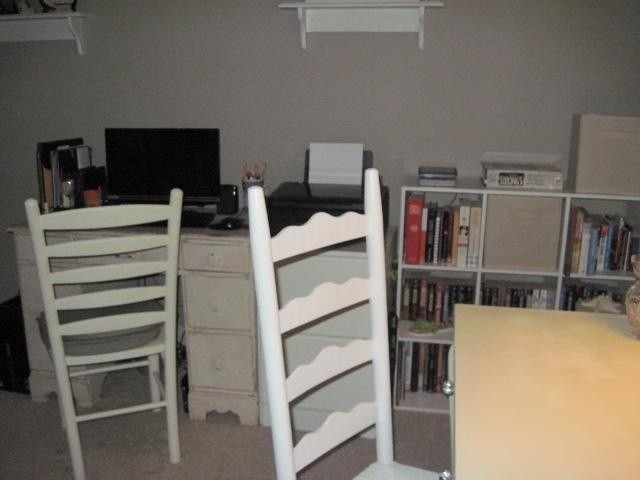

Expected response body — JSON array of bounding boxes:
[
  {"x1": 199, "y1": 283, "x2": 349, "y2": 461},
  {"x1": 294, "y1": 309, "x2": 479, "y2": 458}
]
[{"x1": 268, "y1": 150, "x2": 387, "y2": 242}]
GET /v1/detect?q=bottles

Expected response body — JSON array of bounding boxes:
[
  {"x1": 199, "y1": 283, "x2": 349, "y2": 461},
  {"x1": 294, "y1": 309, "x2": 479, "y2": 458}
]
[{"x1": 626, "y1": 254, "x2": 640, "y2": 342}]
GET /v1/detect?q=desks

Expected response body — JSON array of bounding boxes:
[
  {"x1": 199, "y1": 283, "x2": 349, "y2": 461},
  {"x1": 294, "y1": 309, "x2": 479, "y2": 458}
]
[
  {"x1": 441, "y1": 303, "x2": 639, "y2": 480},
  {"x1": 4, "y1": 202, "x2": 398, "y2": 441}
]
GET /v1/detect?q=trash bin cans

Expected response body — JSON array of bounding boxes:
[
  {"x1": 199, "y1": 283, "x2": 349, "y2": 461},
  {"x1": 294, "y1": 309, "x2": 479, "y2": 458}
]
[{"x1": 0, "y1": 296, "x2": 30, "y2": 395}]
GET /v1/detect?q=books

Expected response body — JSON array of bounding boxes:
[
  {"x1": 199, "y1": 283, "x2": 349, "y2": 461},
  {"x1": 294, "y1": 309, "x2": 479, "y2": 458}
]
[
  {"x1": 418, "y1": 166, "x2": 458, "y2": 179},
  {"x1": 419, "y1": 180, "x2": 458, "y2": 188},
  {"x1": 404, "y1": 193, "x2": 482, "y2": 268},
  {"x1": 480, "y1": 280, "x2": 556, "y2": 309},
  {"x1": 559, "y1": 279, "x2": 629, "y2": 311},
  {"x1": 35, "y1": 143, "x2": 93, "y2": 210},
  {"x1": 478, "y1": 160, "x2": 563, "y2": 190},
  {"x1": 565, "y1": 204, "x2": 633, "y2": 275},
  {"x1": 399, "y1": 341, "x2": 452, "y2": 393},
  {"x1": 401, "y1": 272, "x2": 476, "y2": 323}
]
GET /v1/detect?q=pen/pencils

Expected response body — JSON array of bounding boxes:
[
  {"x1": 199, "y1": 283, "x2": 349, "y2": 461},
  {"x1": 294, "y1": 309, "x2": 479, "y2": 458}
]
[{"x1": 243, "y1": 160, "x2": 264, "y2": 180}]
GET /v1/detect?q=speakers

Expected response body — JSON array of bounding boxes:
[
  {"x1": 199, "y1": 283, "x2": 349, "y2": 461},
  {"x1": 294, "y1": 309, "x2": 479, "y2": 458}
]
[{"x1": 217, "y1": 184, "x2": 238, "y2": 214}]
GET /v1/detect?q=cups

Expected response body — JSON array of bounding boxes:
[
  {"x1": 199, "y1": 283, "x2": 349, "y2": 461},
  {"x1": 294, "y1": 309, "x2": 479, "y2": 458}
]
[{"x1": 241, "y1": 177, "x2": 264, "y2": 207}]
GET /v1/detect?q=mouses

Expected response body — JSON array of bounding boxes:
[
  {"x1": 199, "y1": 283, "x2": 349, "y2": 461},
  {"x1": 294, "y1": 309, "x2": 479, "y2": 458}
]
[{"x1": 224, "y1": 218, "x2": 240, "y2": 229}]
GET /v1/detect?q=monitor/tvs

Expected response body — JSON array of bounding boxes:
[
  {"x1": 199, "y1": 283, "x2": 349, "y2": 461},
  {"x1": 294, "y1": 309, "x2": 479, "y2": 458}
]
[{"x1": 102, "y1": 127, "x2": 220, "y2": 204}]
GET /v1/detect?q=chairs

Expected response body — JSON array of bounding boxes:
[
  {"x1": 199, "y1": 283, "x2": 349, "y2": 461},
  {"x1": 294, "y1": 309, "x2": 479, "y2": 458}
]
[
  {"x1": 245, "y1": 167, "x2": 443, "y2": 480},
  {"x1": 25, "y1": 187, "x2": 183, "y2": 480}
]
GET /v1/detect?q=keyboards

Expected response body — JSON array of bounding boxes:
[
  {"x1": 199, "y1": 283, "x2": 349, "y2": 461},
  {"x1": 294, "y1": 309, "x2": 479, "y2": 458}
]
[{"x1": 145, "y1": 209, "x2": 215, "y2": 228}]
